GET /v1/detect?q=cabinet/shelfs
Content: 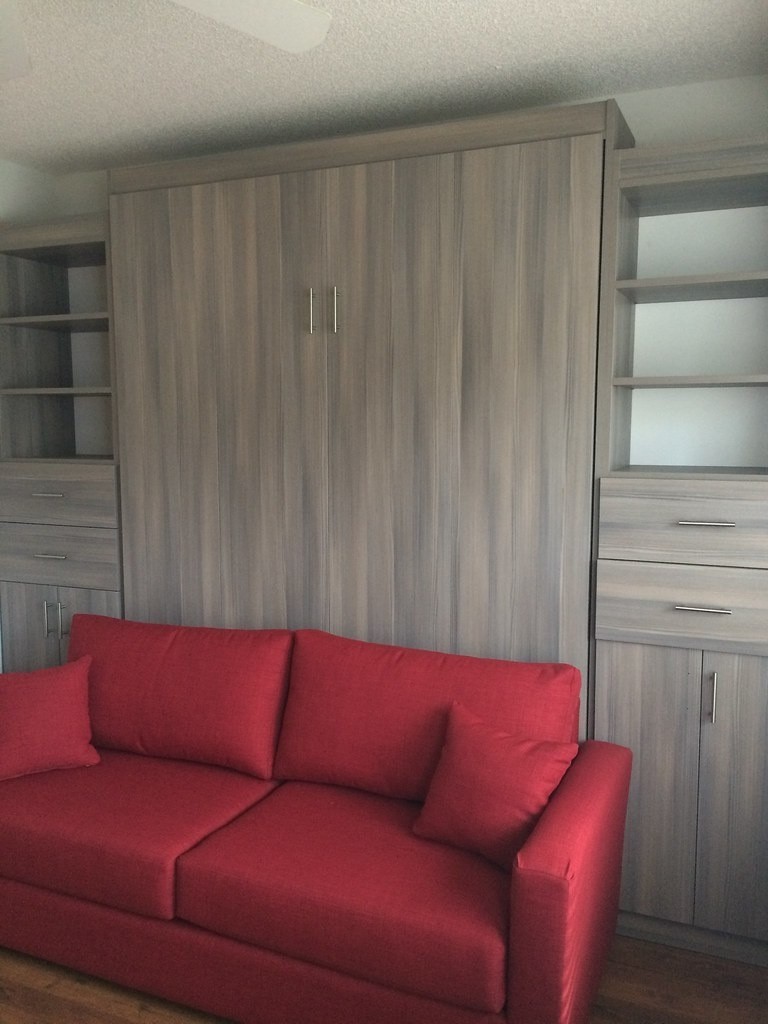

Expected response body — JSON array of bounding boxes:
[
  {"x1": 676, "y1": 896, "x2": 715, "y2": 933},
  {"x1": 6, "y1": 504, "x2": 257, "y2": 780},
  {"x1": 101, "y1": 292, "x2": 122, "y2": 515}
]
[
  {"x1": 588, "y1": 131, "x2": 768, "y2": 981},
  {"x1": 111, "y1": 94, "x2": 639, "y2": 745},
  {"x1": 0, "y1": 207, "x2": 124, "y2": 676}
]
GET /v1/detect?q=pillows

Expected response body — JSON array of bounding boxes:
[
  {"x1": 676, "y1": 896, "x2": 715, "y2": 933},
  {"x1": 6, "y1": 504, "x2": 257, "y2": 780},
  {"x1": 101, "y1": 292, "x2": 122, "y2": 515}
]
[
  {"x1": 69, "y1": 613, "x2": 294, "y2": 779},
  {"x1": 273, "y1": 628, "x2": 581, "y2": 802},
  {"x1": 0, "y1": 654, "x2": 101, "y2": 781},
  {"x1": 411, "y1": 699, "x2": 580, "y2": 871}
]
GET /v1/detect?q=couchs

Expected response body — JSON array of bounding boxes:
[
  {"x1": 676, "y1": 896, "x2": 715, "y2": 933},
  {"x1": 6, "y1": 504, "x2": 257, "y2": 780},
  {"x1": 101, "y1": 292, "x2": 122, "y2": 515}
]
[{"x1": 0, "y1": 612, "x2": 634, "y2": 1024}]
